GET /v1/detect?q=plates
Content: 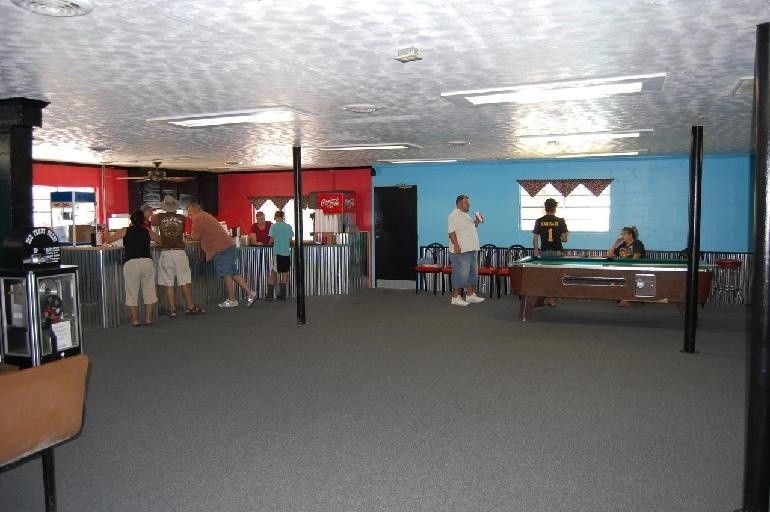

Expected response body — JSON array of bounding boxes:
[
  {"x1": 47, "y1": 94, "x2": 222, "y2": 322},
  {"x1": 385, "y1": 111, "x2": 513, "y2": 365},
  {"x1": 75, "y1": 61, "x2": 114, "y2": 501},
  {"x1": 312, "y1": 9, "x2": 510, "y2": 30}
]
[{"x1": 41, "y1": 295, "x2": 63, "y2": 319}]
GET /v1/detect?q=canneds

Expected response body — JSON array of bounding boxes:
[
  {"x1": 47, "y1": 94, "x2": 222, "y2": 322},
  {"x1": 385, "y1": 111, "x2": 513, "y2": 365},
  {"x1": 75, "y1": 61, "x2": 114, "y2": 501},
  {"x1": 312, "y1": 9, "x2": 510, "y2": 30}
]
[{"x1": 475, "y1": 212, "x2": 484, "y2": 223}]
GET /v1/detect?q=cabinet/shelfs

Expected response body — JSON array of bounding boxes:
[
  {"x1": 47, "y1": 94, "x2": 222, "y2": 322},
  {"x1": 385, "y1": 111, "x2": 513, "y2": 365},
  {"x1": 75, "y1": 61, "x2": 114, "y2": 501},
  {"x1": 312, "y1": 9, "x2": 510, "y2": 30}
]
[{"x1": 1, "y1": 262, "x2": 87, "y2": 369}]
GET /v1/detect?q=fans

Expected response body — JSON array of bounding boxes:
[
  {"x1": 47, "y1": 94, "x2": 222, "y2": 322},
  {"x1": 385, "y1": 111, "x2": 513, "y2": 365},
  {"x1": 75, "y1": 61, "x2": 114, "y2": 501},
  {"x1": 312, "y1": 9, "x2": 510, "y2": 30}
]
[{"x1": 114, "y1": 161, "x2": 197, "y2": 182}]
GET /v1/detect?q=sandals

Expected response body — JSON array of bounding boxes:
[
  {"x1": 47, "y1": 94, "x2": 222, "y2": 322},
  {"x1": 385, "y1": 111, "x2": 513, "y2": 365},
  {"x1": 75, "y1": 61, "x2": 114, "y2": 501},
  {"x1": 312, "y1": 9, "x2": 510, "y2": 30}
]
[
  {"x1": 166, "y1": 309, "x2": 179, "y2": 318},
  {"x1": 184, "y1": 304, "x2": 207, "y2": 315},
  {"x1": 130, "y1": 318, "x2": 156, "y2": 329}
]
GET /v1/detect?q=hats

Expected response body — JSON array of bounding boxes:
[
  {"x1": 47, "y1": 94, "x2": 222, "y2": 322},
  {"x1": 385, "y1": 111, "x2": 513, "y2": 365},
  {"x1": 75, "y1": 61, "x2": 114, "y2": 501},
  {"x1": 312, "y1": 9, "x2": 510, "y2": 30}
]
[
  {"x1": 140, "y1": 204, "x2": 152, "y2": 214},
  {"x1": 157, "y1": 194, "x2": 182, "y2": 212},
  {"x1": 544, "y1": 199, "x2": 560, "y2": 211},
  {"x1": 274, "y1": 211, "x2": 286, "y2": 221}
]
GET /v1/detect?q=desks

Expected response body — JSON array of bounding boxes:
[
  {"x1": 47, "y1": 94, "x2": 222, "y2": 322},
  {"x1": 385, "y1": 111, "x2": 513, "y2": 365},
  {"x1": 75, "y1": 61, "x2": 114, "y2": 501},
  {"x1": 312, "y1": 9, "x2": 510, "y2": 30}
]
[
  {"x1": 1, "y1": 354, "x2": 91, "y2": 511},
  {"x1": 562, "y1": 254, "x2": 609, "y2": 260},
  {"x1": 507, "y1": 255, "x2": 715, "y2": 322}
]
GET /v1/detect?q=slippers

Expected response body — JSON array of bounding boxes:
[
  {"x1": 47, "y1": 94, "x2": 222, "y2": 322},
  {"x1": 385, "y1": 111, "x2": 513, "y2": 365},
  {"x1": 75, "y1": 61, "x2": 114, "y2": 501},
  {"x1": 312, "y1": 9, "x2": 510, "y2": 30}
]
[{"x1": 534, "y1": 301, "x2": 557, "y2": 307}]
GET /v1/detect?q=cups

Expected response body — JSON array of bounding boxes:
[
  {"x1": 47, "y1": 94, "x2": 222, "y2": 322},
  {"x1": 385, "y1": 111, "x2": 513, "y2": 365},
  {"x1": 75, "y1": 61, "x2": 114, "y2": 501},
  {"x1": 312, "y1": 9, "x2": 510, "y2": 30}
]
[
  {"x1": 224, "y1": 227, "x2": 257, "y2": 248},
  {"x1": 475, "y1": 211, "x2": 483, "y2": 224},
  {"x1": 91, "y1": 233, "x2": 96, "y2": 246}
]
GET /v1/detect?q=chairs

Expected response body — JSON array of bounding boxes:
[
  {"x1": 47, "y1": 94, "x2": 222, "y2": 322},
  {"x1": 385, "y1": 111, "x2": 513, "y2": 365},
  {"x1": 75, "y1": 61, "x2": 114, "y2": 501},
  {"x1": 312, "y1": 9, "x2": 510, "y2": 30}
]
[
  {"x1": 677, "y1": 247, "x2": 706, "y2": 308},
  {"x1": 477, "y1": 243, "x2": 501, "y2": 298},
  {"x1": 441, "y1": 264, "x2": 464, "y2": 297},
  {"x1": 414, "y1": 243, "x2": 446, "y2": 295},
  {"x1": 497, "y1": 244, "x2": 528, "y2": 301}
]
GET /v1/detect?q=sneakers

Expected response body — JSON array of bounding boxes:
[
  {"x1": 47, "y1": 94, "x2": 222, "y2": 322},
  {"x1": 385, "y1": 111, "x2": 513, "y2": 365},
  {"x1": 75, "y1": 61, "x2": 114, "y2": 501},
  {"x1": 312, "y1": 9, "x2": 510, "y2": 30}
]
[
  {"x1": 617, "y1": 300, "x2": 631, "y2": 307},
  {"x1": 449, "y1": 296, "x2": 471, "y2": 309},
  {"x1": 465, "y1": 292, "x2": 486, "y2": 306},
  {"x1": 246, "y1": 290, "x2": 259, "y2": 308},
  {"x1": 266, "y1": 291, "x2": 292, "y2": 303},
  {"x1": 217, "y1": 297, "x2": 240, "y2": 310}
]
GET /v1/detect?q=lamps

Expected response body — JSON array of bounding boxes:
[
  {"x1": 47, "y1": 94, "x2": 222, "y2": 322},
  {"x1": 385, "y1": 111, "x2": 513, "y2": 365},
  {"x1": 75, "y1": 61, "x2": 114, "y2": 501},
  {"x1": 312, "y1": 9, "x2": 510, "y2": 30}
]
[
  {"x1": 303, "y1": 141, "x2": 425, "y2": 151},
  {"x1": 514, "y1": 126, "x2": 655, "y2": 140},
  {"x1": 439, "y1": 70, "x2": 667, "y2": 111},
  {"x1": 393, "y1": 44, "x2": 424, "y2": 65},
  {"x1": 544, "y1": 148, "x2": 649, "y2": 159},
  {"x1": 142, "y1": 102, "x2": 320, "y2": 128},
  {"x1": 376, "y1": 156, "x2": 465, "y2": 165}
]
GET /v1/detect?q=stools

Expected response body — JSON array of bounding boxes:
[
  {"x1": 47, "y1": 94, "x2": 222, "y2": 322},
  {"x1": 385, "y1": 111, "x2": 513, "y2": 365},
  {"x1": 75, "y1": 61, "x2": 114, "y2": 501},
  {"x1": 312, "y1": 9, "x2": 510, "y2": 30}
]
[{"x1": 712, "y1": 258, "x2": 744, "y2": 309}]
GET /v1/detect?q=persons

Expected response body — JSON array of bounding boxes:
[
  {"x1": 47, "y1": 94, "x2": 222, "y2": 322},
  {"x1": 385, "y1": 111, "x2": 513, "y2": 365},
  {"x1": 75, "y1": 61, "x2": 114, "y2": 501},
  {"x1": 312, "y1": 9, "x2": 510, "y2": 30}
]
[
  {"x1": 251, "y1": 211, "x2": 272, "y2": 247},
  {"x1": 607, "y1": 227, "x2": 646, "y2": 306},
  {"x1": 449, "y1": 195, "x2": 486, "y2": 306},
  {"x1": 532, "y1": 198, "x2": 568, "y2": 304},
  {"x1": 148, "y1": 194, "x2": 207, "y2": 320},
  {"x1": 105, "y1": 210, "x2": 163, "y2": 326},
  {"x1": 140, "y1": 204, "x2": 157, "y2": 231},
  {"x1": 266, "y1": 211, "x2": 294, "y2": 301},
  {"x1": 184, "y1": 203, "x2": 258, "y2": 309}
]
